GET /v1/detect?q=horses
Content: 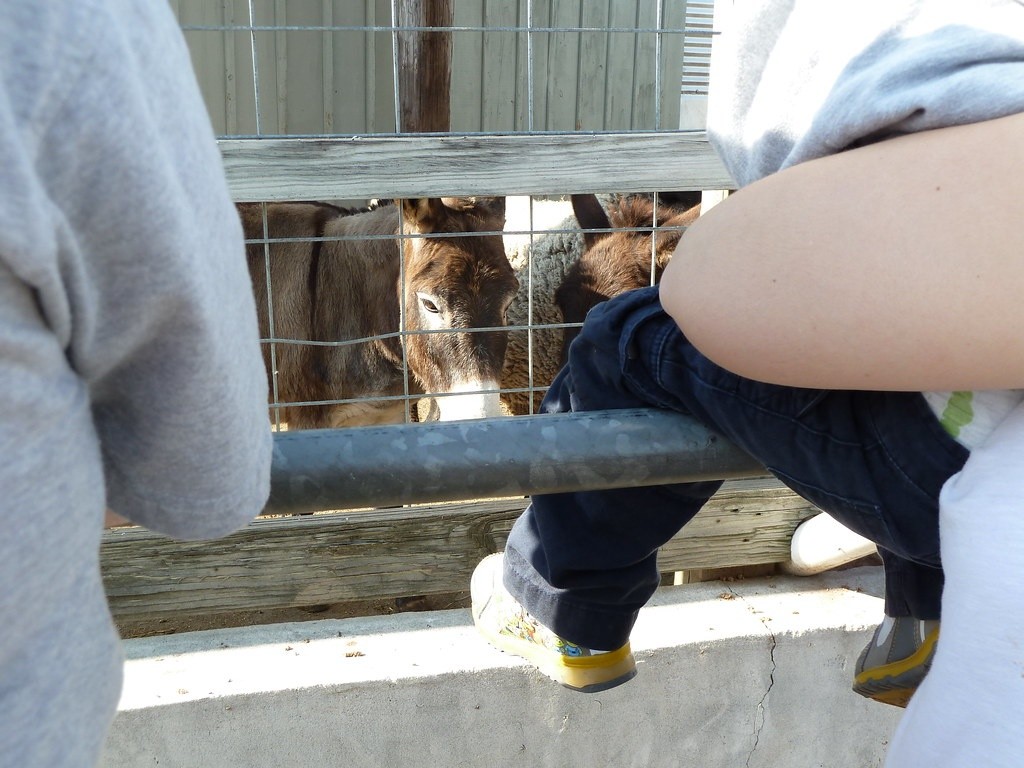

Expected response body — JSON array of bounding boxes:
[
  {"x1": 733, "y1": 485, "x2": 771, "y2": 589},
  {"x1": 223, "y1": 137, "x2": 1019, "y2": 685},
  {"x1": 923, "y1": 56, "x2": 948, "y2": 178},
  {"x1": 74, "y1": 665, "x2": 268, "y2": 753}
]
[
  {"x1": 498, "y1": 191, "x2": 701, "y2": 414},
  {"x1": 234, "y1": 195, "x2": 521, "y2": 433}
]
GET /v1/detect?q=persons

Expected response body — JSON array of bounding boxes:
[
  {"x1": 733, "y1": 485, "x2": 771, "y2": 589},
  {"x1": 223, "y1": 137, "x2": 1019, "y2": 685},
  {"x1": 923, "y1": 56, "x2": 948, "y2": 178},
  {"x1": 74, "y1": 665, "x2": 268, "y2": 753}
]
[
  {"x1": 0, "y1": 0, "x2": 273, "y2": 768},
  {"x1": 468, "y1": 0, "x2": 1024, "y2": 768}
]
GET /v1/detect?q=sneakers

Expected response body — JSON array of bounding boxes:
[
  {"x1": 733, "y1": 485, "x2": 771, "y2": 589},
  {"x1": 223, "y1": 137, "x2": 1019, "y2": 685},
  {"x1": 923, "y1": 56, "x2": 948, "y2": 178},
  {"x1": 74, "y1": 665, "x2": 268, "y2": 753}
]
[
  {"x1": 855, "y1": 615, "x2": 942, "y2": 709},
  {"x1": 471, "y1": 550, "x2": 637, "y2": 693}
]
